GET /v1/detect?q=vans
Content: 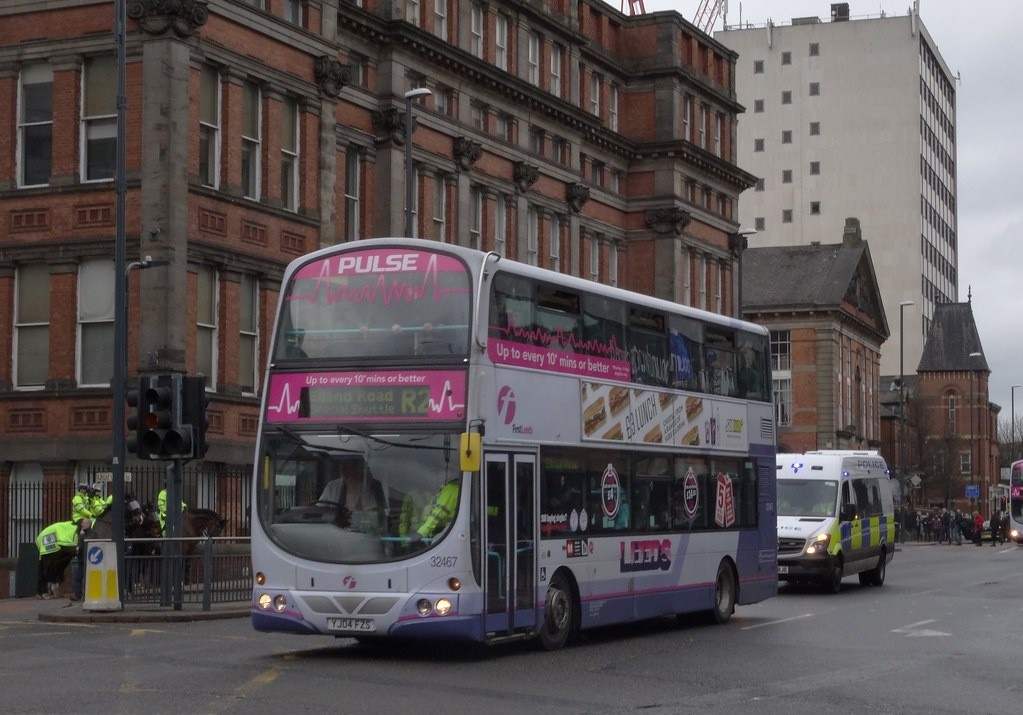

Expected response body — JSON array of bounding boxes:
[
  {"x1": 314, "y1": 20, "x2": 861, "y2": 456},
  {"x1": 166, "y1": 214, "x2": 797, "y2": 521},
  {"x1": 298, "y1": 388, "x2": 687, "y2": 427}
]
[{"x1": 773, "y1": 451, "x2": 896, "y2": 595}]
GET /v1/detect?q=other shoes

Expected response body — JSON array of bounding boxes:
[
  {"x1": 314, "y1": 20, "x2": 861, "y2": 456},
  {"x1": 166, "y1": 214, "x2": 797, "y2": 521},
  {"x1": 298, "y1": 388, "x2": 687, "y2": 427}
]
[
  {"x1": 36, "y1": 593, "x2": 49, "y2": 599},
  {"x1": 71, "y1": 592, "x2": 81, "y2": 601},
  {"x1": 51, "y1": 583, "x2": 59, "y2": 598}
]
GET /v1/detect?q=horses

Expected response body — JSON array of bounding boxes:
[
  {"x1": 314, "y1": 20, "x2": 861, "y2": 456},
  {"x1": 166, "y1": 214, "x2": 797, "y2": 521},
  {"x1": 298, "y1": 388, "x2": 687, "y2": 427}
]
[{"x1": 37, "y1": 495, "x2": 230, "y2": 600}]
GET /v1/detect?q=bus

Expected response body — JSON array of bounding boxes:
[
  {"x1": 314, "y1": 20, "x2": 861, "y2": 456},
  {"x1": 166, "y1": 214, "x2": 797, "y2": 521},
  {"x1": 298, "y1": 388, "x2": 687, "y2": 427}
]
[
  {"x1": 1010, "y1": 459, "x2": 1023, "y2": 542},
  {"x1": 249, "y1": 237, "x2": 780, "y2": 654}
]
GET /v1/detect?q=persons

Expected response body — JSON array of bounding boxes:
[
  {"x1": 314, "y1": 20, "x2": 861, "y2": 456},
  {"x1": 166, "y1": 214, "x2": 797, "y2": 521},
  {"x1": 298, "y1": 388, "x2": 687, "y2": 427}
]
[
  {"x1": 68, "y1": 483, "x2": 106, "y2": 601},
  {"x1": 398, "y1": 458, "x2": 460, "y2": 548},
  {"x1": 915, "y1": 508, "x2": 1010, "y2": 546},
  {"x1": 315, "y1": 459, "x2": 377, "y2": 515},
  {"x1": 157, "y1": 481, "x2": 187, "y2": 537}
]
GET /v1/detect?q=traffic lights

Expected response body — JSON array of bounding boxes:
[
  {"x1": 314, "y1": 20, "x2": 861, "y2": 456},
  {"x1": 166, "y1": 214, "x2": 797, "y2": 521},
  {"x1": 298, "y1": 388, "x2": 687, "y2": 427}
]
[
  {"x1": 1001, "y1": 496, "x2": 1006, "y2": 511},
  {"x1": 180, "y1": 375, "x2": 209, "y2": 459},
  {"x1": 127, "y1": 376, "x2": 151, "y2": 460},
  {"x1": 145, "y1": 370, "x2": 195, "y2": 461}
]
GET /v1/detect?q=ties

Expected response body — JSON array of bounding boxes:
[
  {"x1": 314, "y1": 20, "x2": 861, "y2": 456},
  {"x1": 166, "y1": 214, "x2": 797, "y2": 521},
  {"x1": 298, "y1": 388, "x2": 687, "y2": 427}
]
[{"x1": 335, "y1": 479, "x2": 347, "y2": 522}]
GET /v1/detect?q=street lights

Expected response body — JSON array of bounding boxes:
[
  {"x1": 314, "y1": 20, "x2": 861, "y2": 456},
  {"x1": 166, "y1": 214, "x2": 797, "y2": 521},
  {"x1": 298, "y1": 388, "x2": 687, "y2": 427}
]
[
  {"x1": 1011, "y1": 384, "x2": 1022, "y2": 463},
  {"x1": 898, "y1": 299, "x2": 915, "y2": 543},
  {"x1": 969, "y1": 352, "x2": 982, "y2": 519},
  {"x1": 405, "y1": 86, "x2": 433, "y2": 239}
]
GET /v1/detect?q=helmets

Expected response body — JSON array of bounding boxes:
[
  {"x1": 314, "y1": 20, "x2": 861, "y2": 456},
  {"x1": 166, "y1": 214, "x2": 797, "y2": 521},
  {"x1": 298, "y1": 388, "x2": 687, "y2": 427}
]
[
  {"x1": 78, "y1": 481, "x2": 89, "y2": 491},
  {"x1": 93, "y1": 483, "x2": 102, "y2": 491}
]
